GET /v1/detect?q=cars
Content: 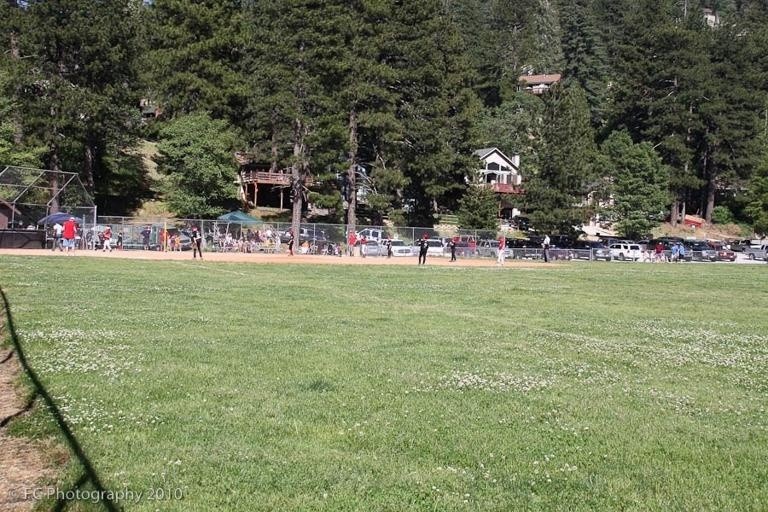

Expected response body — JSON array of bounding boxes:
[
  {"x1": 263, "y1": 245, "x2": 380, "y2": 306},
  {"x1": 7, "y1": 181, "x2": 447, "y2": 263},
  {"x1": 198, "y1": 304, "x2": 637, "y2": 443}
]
[{"x1": 88, "y1": 223, "x2": 191, "y2": 251}]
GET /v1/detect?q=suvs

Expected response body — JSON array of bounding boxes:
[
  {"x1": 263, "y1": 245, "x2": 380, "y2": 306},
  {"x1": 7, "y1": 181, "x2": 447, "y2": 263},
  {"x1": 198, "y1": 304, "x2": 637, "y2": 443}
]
[
  {"x1": 277, "y1": 226, "x2": 327, "y2": 246},
  {"x1": 355, "y1": 227, "x2": 768, "y2": 262}
]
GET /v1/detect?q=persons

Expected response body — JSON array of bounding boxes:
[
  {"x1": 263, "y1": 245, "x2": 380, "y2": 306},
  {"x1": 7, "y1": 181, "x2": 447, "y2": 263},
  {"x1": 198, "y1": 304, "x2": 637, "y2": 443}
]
[{"x1": 49, "y1": 216, "x2": 686, "y2": 265}]
